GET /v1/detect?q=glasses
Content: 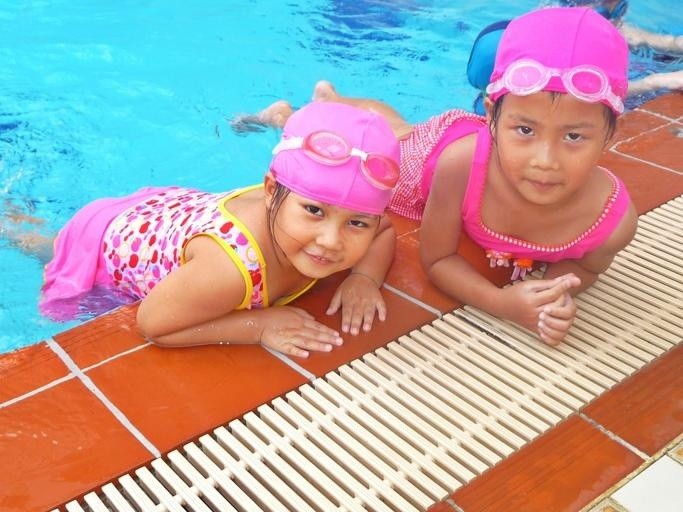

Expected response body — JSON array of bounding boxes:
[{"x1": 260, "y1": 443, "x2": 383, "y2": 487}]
[
  {"x1": 272, "y1": 130, "x2": 400, "y2": 191},
  {"x1": 486, "y1": 58, "x2": 624, "y2": 113}
]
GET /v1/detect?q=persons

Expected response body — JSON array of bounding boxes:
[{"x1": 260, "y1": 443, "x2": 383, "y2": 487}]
[
  {"x1": 8, "y1": 100, "x2": 402, "y2": 359},
  {"x1": 243, "y1": 5, "x2": 638, "y2": 347},
  {"x1": 466, "y1": 0, "x2": 683, "y2": 116}
]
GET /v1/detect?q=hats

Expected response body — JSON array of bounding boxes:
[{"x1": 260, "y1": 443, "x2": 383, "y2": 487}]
[
  {"x1": 489, "y1": 8, "x2": 628, "y2": 115},
  {"x1": 272, "y1": 102, "x2": 400, "y2": 215}
]
